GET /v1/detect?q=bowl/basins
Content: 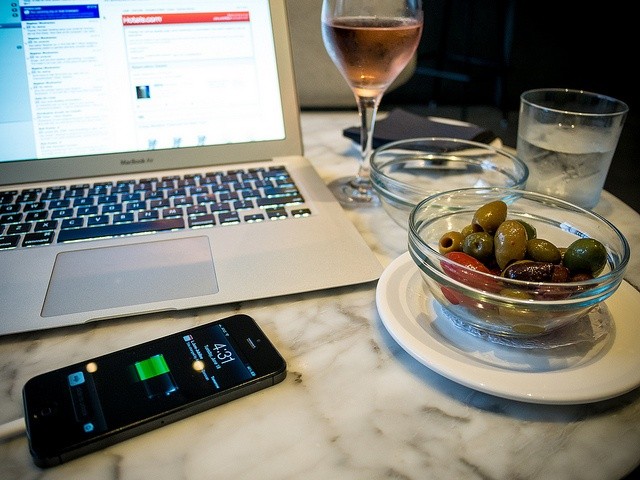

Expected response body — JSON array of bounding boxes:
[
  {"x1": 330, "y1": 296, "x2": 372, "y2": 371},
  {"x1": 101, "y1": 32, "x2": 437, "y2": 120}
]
[
  {"x1": 368, "y1": 136, "x2": 531, "y2": 231},
  {"x1": 405, "y1": 186, "x2": 631, "y2": 340}
]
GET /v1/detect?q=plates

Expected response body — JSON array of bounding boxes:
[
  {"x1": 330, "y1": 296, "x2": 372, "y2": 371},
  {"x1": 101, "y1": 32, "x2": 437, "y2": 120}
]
[{"x1": 374, "y1": 244, "x2": 639, "y2": 407}]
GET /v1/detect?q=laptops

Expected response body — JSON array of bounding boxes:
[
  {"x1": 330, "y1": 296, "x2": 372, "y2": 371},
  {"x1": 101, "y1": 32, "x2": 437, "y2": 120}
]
[{"x1": 0, "y1": 0, "x2": 385, "y2": 336}]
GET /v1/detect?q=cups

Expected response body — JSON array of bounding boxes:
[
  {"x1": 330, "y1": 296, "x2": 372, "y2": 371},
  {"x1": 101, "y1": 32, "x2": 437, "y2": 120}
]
[{"x1": 514, "y1": 86, "x2": 630, "y2": 214}]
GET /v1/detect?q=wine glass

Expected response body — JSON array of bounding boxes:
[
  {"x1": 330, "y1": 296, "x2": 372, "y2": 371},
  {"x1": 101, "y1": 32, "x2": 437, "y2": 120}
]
[{"x1": 319, "y1": 0, "x2": 424, "y2": 209}]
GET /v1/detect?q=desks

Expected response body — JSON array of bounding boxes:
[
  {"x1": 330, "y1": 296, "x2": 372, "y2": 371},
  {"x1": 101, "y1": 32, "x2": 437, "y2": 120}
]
[{"x1": 0, "y1": 110, "x2": 640, "y2": 479}]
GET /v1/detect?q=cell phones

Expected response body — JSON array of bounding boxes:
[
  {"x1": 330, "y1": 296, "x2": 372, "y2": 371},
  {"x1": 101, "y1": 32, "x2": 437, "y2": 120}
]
[{"x1": 22, "y1": 314, "x2": 288, "y2": 469}]
